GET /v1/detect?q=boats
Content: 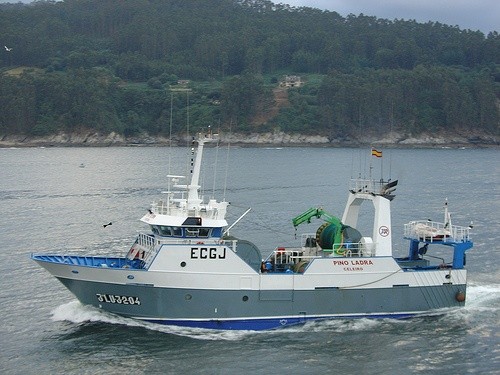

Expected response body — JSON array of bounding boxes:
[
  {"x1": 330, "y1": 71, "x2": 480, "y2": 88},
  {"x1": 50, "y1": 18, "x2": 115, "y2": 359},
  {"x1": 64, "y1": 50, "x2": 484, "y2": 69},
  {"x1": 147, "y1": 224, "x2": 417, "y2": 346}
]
[{"x1": 31, "y1": 86, "x2": 475, "y2": 331}]
